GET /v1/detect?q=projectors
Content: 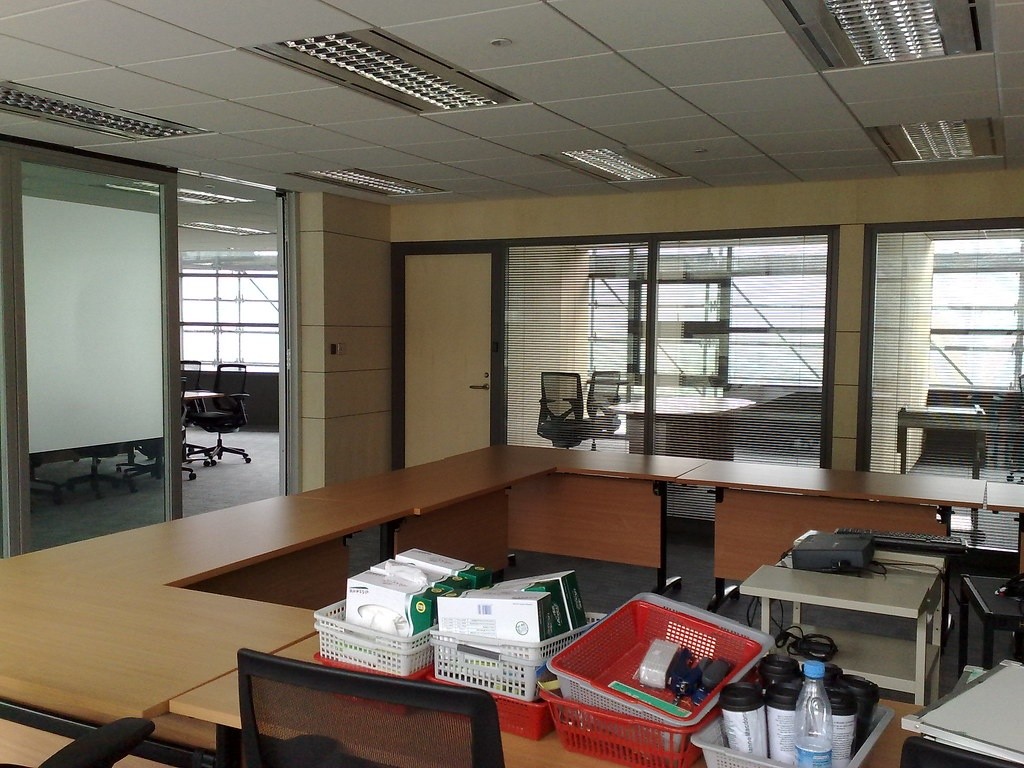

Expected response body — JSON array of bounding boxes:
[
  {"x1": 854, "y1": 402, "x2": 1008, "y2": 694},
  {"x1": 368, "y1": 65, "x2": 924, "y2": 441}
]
[{"x1": 791, "y1": 533, "x2": 875, "y2": 573}]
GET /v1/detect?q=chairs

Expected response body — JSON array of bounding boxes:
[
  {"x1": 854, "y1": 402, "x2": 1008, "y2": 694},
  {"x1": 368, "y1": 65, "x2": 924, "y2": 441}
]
[
  {"x1": 122, "y1": 375, "x2": 196, "y2": 494},
  {"x1": 586, "y1": 371, "x2": 623, "y2": 452},
  {"x1": 237, "y1": 648, "x2": 506, "y2": 768},
  {"x1": 180, "y1": 360, "x2": 210, "y2": 456},
  {"x1": 537, "y1": 372, "x2": 617, "y2": 450},
  {"x1": 66, "y1": 442, "x2": 137, "y2": 500},
  {"x1": 187, "y1": 364, "x2": 252, "y2": 467}
]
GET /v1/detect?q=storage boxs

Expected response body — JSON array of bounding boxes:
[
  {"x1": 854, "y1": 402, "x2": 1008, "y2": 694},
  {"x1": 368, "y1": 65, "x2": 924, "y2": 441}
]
[
  {"x1": 427, "y1": 673, "x2": 554, "y2": 741},
  {"x1": 546, "y1": 592, "x2": 775, "y2": 768},
  {"x1": 370, "y1": 559, "x2": 474, "y2": 597},
  {"x1": 478, "y1": 570, "x2": 587, "y2": 640},
  {"x1": 690, "y1": 689, "x2": 895, "y2": 768},
  {"x1": 314, "y1": 600, "x2": 440, "y2": 650},
  {"x1": 437, "y1": 589, "x2": 552, "y2": 668},
  {"x1": 479, "y1": 579, "x2": 573, "y2": 651},
  {"x1": 539, "y1": 667, "x2": 761, "y2": 768},
  {"x1": 396, "y1": 548, "x2": 493, "y2": 590},
  {"x1": 343, "y1": 570, "x2": 453, "y2": 652},
  {"x1": 430, "y1": 612, "x2": 609, "y2": 684},
  {"x1": 429, "y1": 638, "x2": 556, "y2": 703},
  {"x1": 551, "y1": 600, "x2": 763, "y2": 721},
  {"x1": 313, "y1": 621, "x2": 435, "y2": 676},
  {"x1": 314, "y1": 652, "x2": 434, "y2": 715}
]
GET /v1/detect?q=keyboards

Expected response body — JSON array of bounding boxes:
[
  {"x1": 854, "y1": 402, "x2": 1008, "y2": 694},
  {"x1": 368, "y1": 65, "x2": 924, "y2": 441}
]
[{"x1": 834, "y1": 527, "x2": 968, "y2": 556}]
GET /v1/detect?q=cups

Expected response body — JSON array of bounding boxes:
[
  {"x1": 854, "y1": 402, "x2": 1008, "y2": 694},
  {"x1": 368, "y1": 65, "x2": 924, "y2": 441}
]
[
  {"x1": 824, "y1": 685, "x2": 858, "y2": 768},
  {"x1": 764, "y1": 681, "x2": 801, "y2": 764},
  {"x1": 801, "y1": 661, "x2": 844, "y2": 699},
  {"x1": 837, "y1": 674, "x2": 879, "y2": 755},
  {"x1": 718, "y1": 682, "x2": 768, "y2": 759},
  {"x1": 758, "y1": 653, "x2": 799, "y2": 698}
]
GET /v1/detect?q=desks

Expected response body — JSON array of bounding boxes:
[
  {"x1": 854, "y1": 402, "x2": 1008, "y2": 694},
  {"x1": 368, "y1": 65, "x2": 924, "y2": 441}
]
[
  {"x1": 986, "y1": 482, "x2": 1024, "y2": 577},
  {"x1": 153, "y1": 633, "x2": 922, "y2": 768},
  {"x1": 606, "y1": 397, "x2": 756, "y2": 462},
  {"x1": 445, "y1": 445, "x2": 709, "y2": 598},
  {"x1": 677, "y1": 460, "x2": 986, "y2": 613},
  {"x1": 0, "y1": 559, "x2": 326, "y2": 740},
  {"x1": 8, "y1": 496, "x2": 415, "y2": 611},
  {"x1": 289, "y1": 456, "x2": 558, "y2": 565}
]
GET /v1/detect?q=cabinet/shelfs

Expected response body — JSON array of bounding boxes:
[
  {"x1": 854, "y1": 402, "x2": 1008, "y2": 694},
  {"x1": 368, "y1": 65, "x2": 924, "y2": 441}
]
[{"x1": 740, "y1": 529, "x2": 948, "y2": 707}]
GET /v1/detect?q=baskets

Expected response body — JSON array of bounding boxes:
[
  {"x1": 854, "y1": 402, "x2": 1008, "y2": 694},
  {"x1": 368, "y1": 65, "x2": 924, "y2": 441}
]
[
  {"x1": 427, "y1": 612, "x2": 610, "y2": 740},
  {"x1": 539, "y1": 591, "x2": 775, "y2": 768},
  {"x1": 690, "y1": 700, "x2": 895, "y2": 768},
  {"x1": 313, "y1": 599, "x2": 437, "y2": 715}
]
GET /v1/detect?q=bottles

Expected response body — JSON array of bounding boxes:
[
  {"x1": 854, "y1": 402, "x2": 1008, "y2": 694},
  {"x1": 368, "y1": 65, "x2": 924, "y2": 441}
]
[{"x1": 792, "y1": 659, "x2": 833, "y2": 768}]
